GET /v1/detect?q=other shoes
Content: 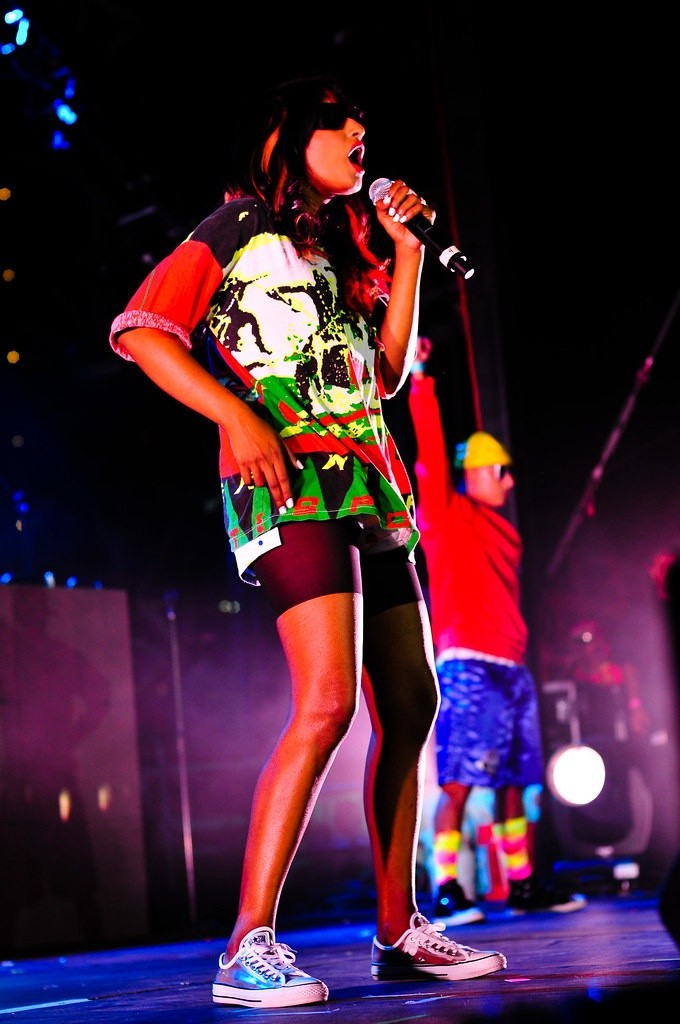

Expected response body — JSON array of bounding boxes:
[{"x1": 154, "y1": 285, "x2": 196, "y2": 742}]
[
  {"x1": 505, "y1": 874, "x2": 585, "y2": 914},
  {"x1": 434, "y1": 882, "x2": 483, "y2": 927}
]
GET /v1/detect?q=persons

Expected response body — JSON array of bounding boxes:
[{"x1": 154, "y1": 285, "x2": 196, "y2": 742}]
[
  {"x1": 106, "y1": 83, "x2": 510, "y2": 1012},
  {"x1": 409, "y1": 332, "x2": 588, "y2": 929}
]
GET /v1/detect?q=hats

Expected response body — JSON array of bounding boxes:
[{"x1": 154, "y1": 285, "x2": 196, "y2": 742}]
[{"x1": 456, "y1": 431, "x2": 513, "y2": 468}]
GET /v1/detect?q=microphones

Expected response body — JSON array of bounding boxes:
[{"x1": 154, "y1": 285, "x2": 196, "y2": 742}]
[{"x1": 368, "y1": 177, "x2": 475, "y2": 279}]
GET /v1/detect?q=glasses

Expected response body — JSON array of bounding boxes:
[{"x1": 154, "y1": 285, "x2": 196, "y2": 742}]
[
  {"x1": 494, "y1": 465, "x2": 507, "y2": 480},
  {"x1": 292, "y1": 97, "x2": 368, "y2": 131}
]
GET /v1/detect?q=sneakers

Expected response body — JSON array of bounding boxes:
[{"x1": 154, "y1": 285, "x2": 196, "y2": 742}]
[
  {"x1": 212, "y1": 926, "x2": 328, "y2": 1009},
  {"x1": 371, "y1": 911, "x2": 508, "y2": 980}
]
